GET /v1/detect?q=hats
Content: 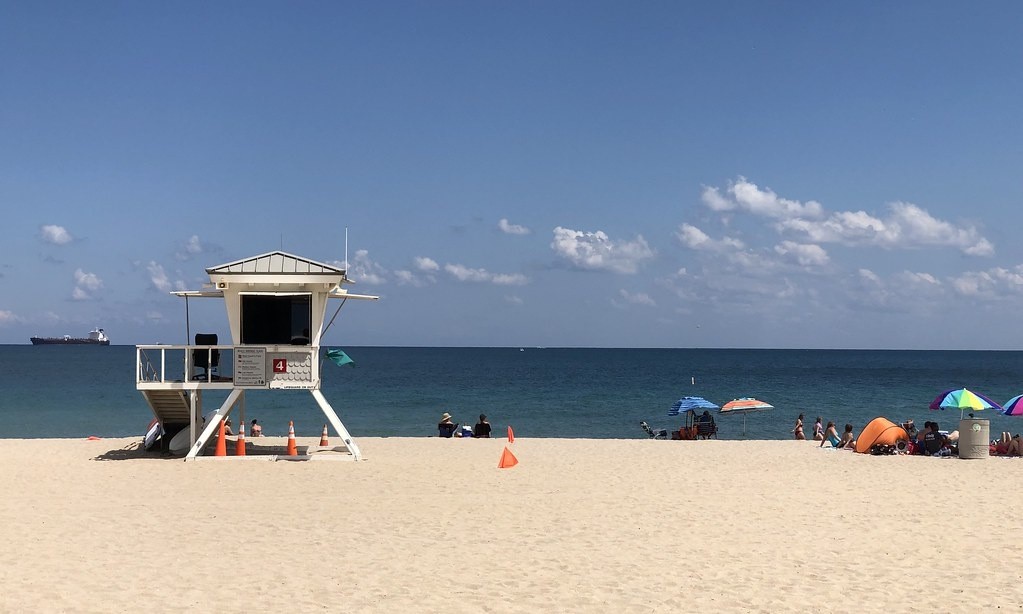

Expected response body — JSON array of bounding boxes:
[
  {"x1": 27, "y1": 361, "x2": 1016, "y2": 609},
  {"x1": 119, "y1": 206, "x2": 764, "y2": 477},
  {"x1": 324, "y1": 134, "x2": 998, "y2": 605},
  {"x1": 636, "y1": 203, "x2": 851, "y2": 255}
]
[
  {"x1": 904, "y1": 420, "x2": 913, "y2": 424},
  {"x1": 441, "y1": 413, "x2": 452, "y2": 422}
]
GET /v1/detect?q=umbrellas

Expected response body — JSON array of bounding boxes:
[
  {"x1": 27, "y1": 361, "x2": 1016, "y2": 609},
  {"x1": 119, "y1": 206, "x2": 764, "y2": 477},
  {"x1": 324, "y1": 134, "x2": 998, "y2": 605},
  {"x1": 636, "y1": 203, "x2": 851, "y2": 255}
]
[
  {"x1": 930, "y1": 386, "x2": 1004, "y2": 419},
  {"x1": 1000, "y1": 393, "x2": 1023, "y2": 416},
  {"x1": 667, "y1": 396, "x2": 721, "y2": 438},
  {"x1": 717, "y1": 397, "x2": 775, "y2": 432}
]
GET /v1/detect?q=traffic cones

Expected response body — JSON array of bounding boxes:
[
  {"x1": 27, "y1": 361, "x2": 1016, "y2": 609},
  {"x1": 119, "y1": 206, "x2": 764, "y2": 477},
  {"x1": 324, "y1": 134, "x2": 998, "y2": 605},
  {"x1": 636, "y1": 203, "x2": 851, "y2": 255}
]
[
  {"x1": 287, "y1": 420, "x2": 297, "y2": 455},
  {"x1": 215, "y1": 420, "x2": 227, "y2": 456},
  {"x1": 317, "y1": 424, "x2": 328, "y2": 452},
  {"x1": 235, "y1": 421, "x2": 245, "y2": 456}
]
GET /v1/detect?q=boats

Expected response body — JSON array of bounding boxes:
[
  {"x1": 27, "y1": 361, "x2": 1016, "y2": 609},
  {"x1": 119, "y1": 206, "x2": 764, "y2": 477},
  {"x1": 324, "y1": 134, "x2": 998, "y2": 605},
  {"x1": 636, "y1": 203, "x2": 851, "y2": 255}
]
[
  {"x1": 168, "y1": 409, "x2": 220, "y2": 455},
  {"x1": 30, "y1": 327, "x2": 111, "y2": 346}
]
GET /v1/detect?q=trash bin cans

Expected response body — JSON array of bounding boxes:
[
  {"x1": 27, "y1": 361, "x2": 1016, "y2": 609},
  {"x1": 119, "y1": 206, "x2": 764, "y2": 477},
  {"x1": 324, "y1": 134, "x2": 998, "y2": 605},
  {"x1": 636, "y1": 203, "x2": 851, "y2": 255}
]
[{"x1": 959, "y1": 418, "x2": 990, "y2": 459}]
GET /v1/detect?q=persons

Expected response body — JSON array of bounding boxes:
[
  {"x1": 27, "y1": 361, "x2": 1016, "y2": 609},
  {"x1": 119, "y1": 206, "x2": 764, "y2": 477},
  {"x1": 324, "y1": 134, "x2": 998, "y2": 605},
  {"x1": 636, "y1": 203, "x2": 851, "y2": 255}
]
[
  {"x1": 702, "y1": 411, "x2": 714, "y2": 438},
  {"x1": 991, "y1": 432, "x2": 1023, "y2": 455},
  {"x1": 475, "y1": 414, "x2": 491, "y2": 438},
  {"x1": 441, "y1": 412, "x2": 454, "y2": 437},
  {"x1": 813, "y1": 416, "x2": 823, "y2": 440},
  {"x1": 918, "y1": 421, "x2": 959, "y2": 457},
  {"x1": 820, "y1": 421, "x2": 845, "y2": 448},
  {"x1": 790, "y1": 413, "x2": 806, "y2": 439},
  {"x1": 225, "y1": 418, "x2": 233, "y2": 435},
  {"x1": 837, "y1": 424, "x2": 856, "y2": 449},
  {"x1": 251, "y1": 419, "x2": 261, "y2": 437}
]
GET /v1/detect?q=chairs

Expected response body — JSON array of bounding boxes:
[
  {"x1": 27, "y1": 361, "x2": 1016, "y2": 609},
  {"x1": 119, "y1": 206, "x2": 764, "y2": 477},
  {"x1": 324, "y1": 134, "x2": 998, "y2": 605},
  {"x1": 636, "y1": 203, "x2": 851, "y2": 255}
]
[
  {"x1": 697, "y1": 422, "x2": 717, "y2": 440},
  {"x1": 192, "y1": 333, "x2": 221, "y2": 380},
  {"x1": 640, "y1": 422, "x2": 667, "y2": 439}
]
